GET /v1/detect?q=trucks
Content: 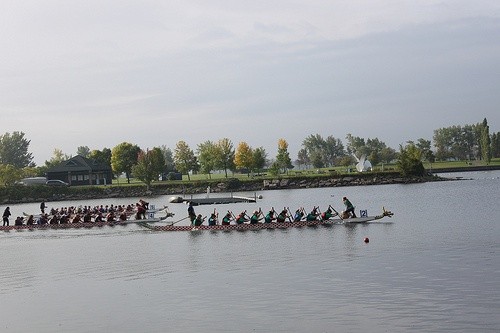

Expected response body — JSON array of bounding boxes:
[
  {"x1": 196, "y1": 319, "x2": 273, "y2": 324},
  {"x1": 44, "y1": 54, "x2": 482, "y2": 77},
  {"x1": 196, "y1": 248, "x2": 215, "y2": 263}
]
[{"x1": 18, "y1": 177, "x2": 47, "y2": 185}]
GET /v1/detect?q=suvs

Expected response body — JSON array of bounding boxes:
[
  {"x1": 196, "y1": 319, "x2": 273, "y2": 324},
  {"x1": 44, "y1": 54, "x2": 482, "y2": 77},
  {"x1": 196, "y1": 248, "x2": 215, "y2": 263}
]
[{"x1": 46, "y1": 180, "x2": 70, "y2": 187}]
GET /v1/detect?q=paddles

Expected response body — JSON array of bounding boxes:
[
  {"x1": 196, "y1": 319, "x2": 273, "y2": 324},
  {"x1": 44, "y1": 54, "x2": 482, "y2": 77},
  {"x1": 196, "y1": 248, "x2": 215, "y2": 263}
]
[
  {"x1": 203, "y1": 216, "x2": 207, "y2": 219},
  {"x1": 329, "y1": 204, "x2": 343, "y2": 220},
  {"x1": 286, "y1": 210, "x2": 292, "y2": 223},
  {"x1": 303, "y1": 208, "x2": 307, "y2": 215},
  {"x1": 167, "y1": 216, "x2": 190, "y2": 226},
  {"x1": 314, "y1": 206, "x2": 322, "y2": 220},
  {"x1": 287, "y1": 207, "x2": 294, "y2": 220},
  {"x1": 318, "y1": 207, "x2": 321, "y2": 215},
  {"x1": 244, "y1": 208, "x2": 266, "y2": 221},
  {"x1": 217, "y1": 213, "x2": 218, "y2": 226},
  {"x1": 275, "y1": 213, "x2": 278, "y2": 219},
  {"x1": 228, "y1": 210, "x2": 236, "y2": 223},
  {"x1": 272, "y1": 207, "x2": 279, "y2": 216},
  {"x1": 231, "y1": 212, "x2": 236, "y2": 219},
  {"x1": 300, "y1": 207, "x2": 305, "y2": 217},
  {"x1": 214, "y1": 208, "x2": 215, "y2": 215}
]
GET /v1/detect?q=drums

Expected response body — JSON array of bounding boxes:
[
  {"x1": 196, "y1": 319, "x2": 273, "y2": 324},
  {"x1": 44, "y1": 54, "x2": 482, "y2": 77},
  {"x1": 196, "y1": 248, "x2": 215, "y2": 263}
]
[{"x1": 342, "y1": 211, "x2": 348, "y2": 219}]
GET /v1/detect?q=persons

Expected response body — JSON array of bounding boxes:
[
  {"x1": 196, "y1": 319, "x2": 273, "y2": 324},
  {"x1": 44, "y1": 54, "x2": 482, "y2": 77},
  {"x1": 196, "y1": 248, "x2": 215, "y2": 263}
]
[
  {"x1": 138, "y1": 198, "x2": 149, "y2": 210},
  {"x1": 188, "y1": 201, "x2": 196, "y2": 227},
  {"x1": 136, "y1": 203, "x2": 145, "y2": 219},
  {"x1": 15, "y1": 203, "x2": 135, "y2": 226},
  {"x1": 192, "y1": 205, "x2": 339, "y2": 226},
  {"x1": 2, "y1": 207, "x2": 11, "y2": 227},
  {"x1": 39, "y1": 200, "x2": 48, "y2": 213},
  {"x1": 207, "y1": 185, "x2": 211, "y2": 198},
  {"x1": 342, "y1": 196, "x2": 356, "y2": 218}
]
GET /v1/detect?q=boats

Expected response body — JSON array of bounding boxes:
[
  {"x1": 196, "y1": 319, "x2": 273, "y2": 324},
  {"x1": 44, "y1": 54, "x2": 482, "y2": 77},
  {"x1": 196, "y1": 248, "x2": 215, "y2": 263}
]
[
  {"x1": 0, "y1": 205, "x2": 176, "y2": 231},
  {"x1": 138, "y1": 206, "x2": 394, "y2": 231}
]
